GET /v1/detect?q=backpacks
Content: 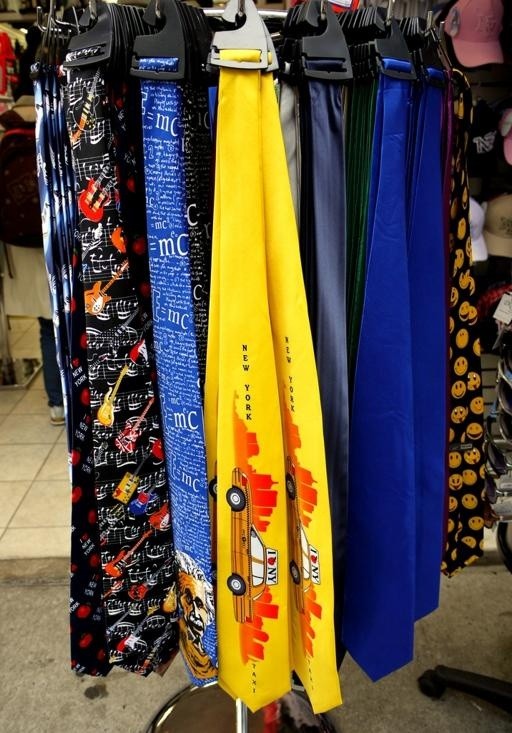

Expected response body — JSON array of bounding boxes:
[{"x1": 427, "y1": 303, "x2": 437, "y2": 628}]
[{"x1": 1, "y1": 111, "x2": 43, "y2": 278}]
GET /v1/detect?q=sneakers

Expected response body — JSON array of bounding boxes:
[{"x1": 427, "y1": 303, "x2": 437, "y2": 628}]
[{"x1": 48, "y1": 404, "x2": 66, "y2": 424}]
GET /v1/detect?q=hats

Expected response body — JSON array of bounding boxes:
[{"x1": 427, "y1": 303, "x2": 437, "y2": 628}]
[
  {"x1": 464, "y1": 96, "x2": 496, "y2": 177},
  {"x1": 443, "y1": 0, "x2": 506, "y2": 67},
  {"x1": 467, "y1": 203, "x2": 488, "y2": 263},
  {"x1": 485, "y1": 193, "x2": 512, "y2": 260}
]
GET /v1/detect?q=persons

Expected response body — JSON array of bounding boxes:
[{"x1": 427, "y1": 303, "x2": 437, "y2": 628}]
[{"x1": 0, "y1": 48, "x2": 73, "y2": 427}]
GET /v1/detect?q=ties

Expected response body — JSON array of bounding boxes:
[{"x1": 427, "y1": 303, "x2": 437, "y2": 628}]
[
  {"x1": 250, "y1": 52, "x2": 344, "y2": 717},
  {"x1": 65, "y1": 200, "x2": 119, "y2": 681},
  {"x1": 351, "y1": 57, "x2": 416, "y2": 686},
  {"x1": 139, "y1": 52, "x2": 222, "y2": 685},
  {"x1": 210, "y1": 47, "x2": 310, "y2": 665},
  {"x1": 309, "y1": 75, "x2": 358, "y2": 677},
  {"x1": 444, "y1": 68, "x2": 488, "y2": 578},
  {"x1": 32, "y1": 61, "x2": 71, "y2": 405},
  {"x1": 413, "y1": 64, "x2": 441, "y2": 616},
  {"x1": 71, "y1": 47, "x2": 179, "y2": 682},
  {"x1": 273, "y1": 74, "x2": 301, "y2": 220}
]
